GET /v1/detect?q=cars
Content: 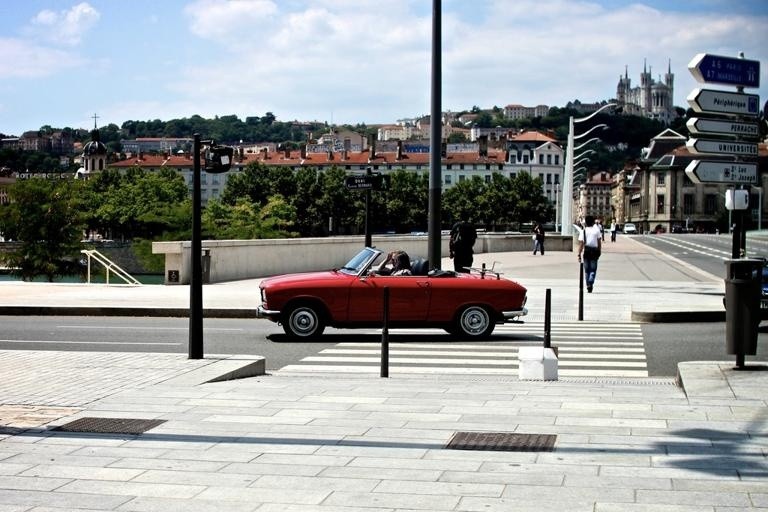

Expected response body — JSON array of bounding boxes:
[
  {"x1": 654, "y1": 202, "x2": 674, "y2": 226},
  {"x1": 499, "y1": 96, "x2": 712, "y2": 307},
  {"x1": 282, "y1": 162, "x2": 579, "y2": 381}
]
[{"x1": 624, "y1": 223, "x2": 637, "y2": 234}]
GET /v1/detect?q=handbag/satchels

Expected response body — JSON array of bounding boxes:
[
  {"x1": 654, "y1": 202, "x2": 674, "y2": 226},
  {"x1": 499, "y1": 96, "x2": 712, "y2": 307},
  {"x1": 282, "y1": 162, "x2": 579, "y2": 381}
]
[
  {"x1": 452, "y1": 224, "x2": 465, "y2": 247},
  {"x1": 532, "y1": 234, "x2": 539, "y2": 240},
  {"x1": 584, "y1": 246, "x2": 600, "y2": 260}
]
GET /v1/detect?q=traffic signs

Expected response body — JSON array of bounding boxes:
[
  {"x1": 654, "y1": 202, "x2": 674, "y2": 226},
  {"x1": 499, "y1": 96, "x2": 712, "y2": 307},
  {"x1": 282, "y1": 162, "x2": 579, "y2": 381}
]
[{"x1": 685, "y1": 49, "x2": 759, "y2": 183}]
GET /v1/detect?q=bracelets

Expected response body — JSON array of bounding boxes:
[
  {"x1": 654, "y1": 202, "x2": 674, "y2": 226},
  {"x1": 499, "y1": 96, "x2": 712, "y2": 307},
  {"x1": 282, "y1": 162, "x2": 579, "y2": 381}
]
[{"x1": 577, "y1": 255, "x2": 581, "y2": 257}]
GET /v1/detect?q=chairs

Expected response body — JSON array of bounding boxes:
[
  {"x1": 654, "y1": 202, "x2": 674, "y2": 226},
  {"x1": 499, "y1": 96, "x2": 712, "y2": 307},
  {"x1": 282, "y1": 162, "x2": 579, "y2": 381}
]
[{"x1": 410, "y1": 256, "x2": 429, "y2": 275}]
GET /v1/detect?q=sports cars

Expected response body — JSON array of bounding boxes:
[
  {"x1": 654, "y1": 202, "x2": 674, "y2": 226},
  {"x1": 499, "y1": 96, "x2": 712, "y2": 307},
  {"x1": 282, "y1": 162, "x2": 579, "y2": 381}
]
[{"x1": 256, "y1": 247, "x2": 529, "y2": 340}]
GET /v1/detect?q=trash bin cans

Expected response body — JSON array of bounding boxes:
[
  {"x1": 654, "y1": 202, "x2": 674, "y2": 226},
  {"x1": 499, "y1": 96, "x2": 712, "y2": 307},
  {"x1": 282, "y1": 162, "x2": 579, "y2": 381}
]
[
  {"x1": 200, "y1": 248, "x2": 211, "y2": 283},
  {"x1": 723, "y1": 258, "x2": 764, "y2": 355}
]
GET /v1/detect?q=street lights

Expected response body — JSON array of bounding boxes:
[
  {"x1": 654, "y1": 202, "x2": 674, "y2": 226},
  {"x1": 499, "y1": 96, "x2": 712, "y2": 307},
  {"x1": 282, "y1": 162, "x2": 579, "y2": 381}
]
[{"x1": 560, "y1": 101, "x2": 623, "y2": 234}]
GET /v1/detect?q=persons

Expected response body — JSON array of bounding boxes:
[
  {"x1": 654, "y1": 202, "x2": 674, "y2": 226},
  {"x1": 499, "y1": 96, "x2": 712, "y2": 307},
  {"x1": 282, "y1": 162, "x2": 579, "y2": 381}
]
[
  {"x1": 577, "y1": 215, "x2": 602, "y2": 293},
  {"x1": 610, "y1": 219, "x2": 617, "y2": 242},
  {"x1": 577, "y1": 213, "x2": 604, "y2": 242},
  {"x1": 448, "y1": 211, "x2": 477, "y2": 273},
  {"x1": 531, "y1": 220, "x2": 545, "y2": 255},
  {"x1": 373, "y1": 250, "x2": 413, "y2": 276}
]
